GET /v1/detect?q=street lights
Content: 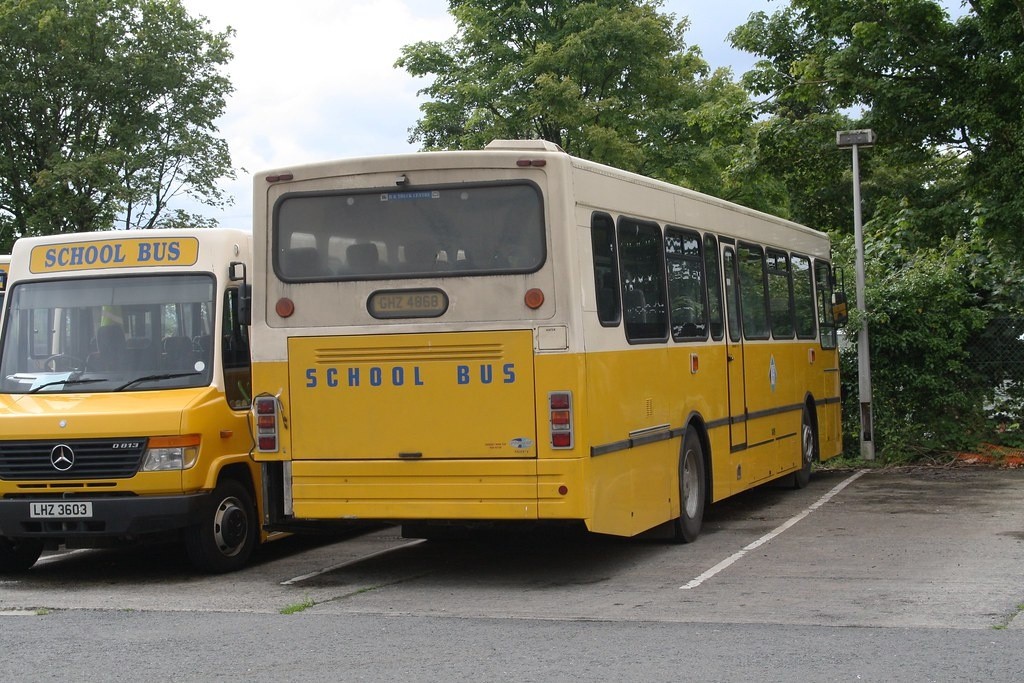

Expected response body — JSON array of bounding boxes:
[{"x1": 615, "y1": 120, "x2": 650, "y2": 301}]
[{"x1": 837, "y1": 129, "x2": 878, "y2": 463}]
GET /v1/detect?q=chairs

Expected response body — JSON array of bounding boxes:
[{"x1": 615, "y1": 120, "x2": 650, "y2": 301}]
[{"x1": 86, "y1": 325, "x2": 144, "y2": 371}]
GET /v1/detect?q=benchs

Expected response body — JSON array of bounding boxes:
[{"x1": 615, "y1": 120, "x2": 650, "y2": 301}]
[
  {"x1": 87, "y1": 333, "x2": 248, "y2": 370},
  {"x1": 600, "y1": 287, "x2": 771, "y2": 337},
  {"x1": 285, "y1": 239, "x2": 521, "y2": 276}
]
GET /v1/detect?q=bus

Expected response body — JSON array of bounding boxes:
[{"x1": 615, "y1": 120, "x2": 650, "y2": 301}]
[
  {"x1": 250, "y1": 139, "x2": 848, "y2": 543},
  {"x1": 0, "y1": 227, "x2": 464, "y2": 576}
]
